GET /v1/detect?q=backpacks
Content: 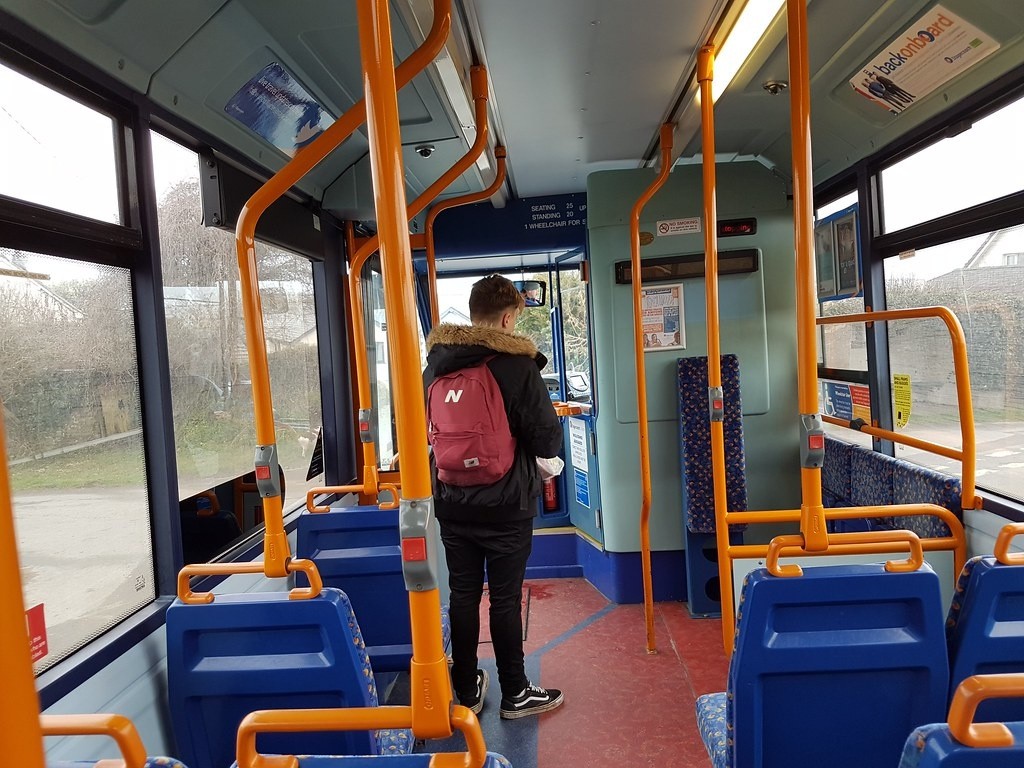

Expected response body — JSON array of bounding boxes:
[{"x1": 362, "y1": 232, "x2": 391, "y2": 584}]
[{"x1": 426, "y1": 354, "x2": 517, "y2": 487}]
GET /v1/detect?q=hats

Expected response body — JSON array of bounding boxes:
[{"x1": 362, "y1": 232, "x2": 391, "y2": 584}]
[{"x1": 523, "y1": 282, "x2": 540, "y2": 290}]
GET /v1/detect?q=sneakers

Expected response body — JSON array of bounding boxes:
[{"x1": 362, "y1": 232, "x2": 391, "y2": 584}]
[
  {"x1": 499, "y1": 679, "x2": 564, "y2": 719},
  {"x1": 460, "y1": 669, "x2": 489, "y2": 714}
]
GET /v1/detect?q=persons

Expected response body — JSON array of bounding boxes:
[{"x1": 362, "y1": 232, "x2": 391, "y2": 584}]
[
  {"x1": 520, "y1": 288, "x2": 530, "y2": 300},
  {"x1": 421, "y1": 272, "x2": 564, "y2": 720}
]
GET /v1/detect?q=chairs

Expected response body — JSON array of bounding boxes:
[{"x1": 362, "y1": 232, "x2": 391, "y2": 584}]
[
  {"x1": 295, "y1": 482, "x2": 450, "y2": 674},
  {"x1": 229, "y1": 704, "x2": 512, "y2": 768},
  {"x1": 898, "y1": 674, "x2": 1024, "y2": 768},
  {"x1": 38, "y1": 714, "x2": 186, "y2": 768},
  {"x1": 695, "y1": 529, "x2": 950, "y2": 768},
  {"x1": 165, "y1": 557, "x2": 426, "y2": 768},
  {"x1": 945, "y1": 522, "x2": 1024, "y2": 723}
]
